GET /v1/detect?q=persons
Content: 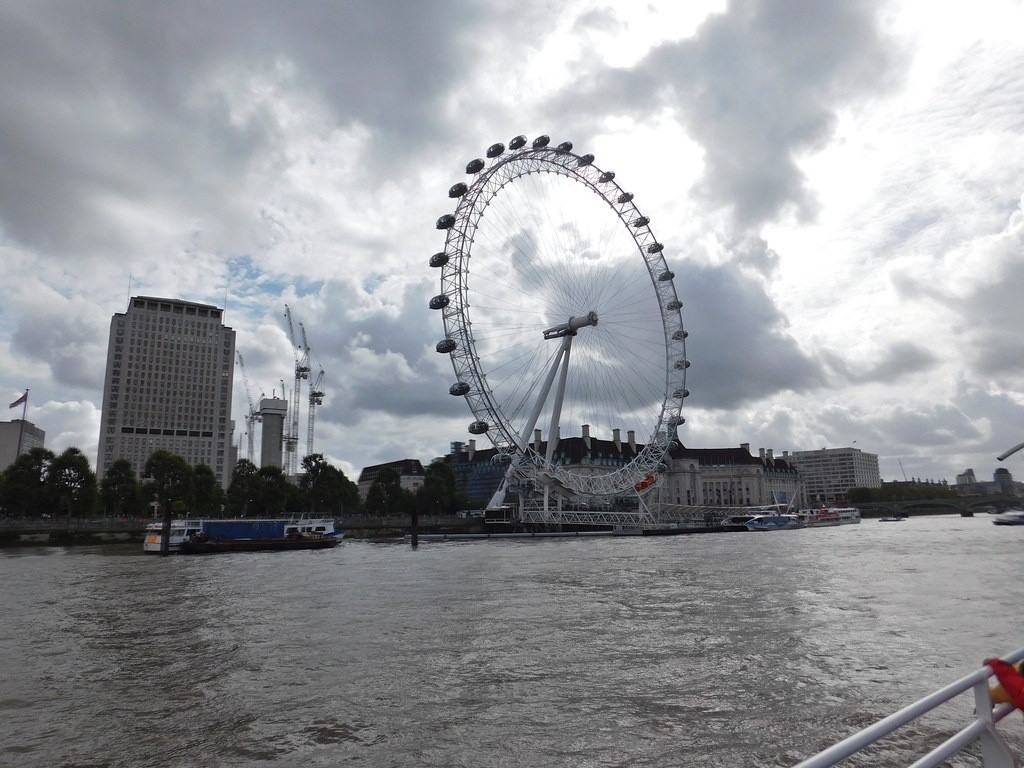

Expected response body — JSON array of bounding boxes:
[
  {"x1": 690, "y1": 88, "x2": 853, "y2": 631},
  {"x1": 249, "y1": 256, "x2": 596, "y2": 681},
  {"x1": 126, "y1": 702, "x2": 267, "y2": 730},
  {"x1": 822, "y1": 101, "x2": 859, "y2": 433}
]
[{"x1": 972, "y1": 657, "x2": 1024, "y2": 717}]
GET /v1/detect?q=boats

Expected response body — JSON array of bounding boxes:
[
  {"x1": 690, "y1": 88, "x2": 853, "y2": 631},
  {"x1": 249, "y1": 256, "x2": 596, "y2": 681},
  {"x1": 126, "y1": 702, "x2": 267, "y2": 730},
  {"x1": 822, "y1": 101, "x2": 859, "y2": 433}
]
[
  {"x1": 142, "y1": 510, "x2": 345, "y2": 553},
  {"x1": 741, "y1": 507, "x2": 862, "y2": 532},
  {"x1": 991, "y1": 510, "x2": 1024, "y2": 526}
]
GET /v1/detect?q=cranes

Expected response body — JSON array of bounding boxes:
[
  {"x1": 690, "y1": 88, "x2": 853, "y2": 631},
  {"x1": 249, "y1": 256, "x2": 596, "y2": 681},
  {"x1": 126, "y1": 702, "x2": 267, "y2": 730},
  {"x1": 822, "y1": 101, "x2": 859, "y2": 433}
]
[
  {"x1": 281, "y1": 304, "x2": 327, "y2": 477},
  {"x1": 235, "y1": 350, "x2": 267, "y2": 464}
]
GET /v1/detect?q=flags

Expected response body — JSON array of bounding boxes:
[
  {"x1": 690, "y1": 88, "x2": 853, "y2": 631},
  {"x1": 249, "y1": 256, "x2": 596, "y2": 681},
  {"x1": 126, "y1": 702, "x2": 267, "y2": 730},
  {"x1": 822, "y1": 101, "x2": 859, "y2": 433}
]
[{"x1": 9, "y1": 392, "x2": 27, "y2": 408}]
